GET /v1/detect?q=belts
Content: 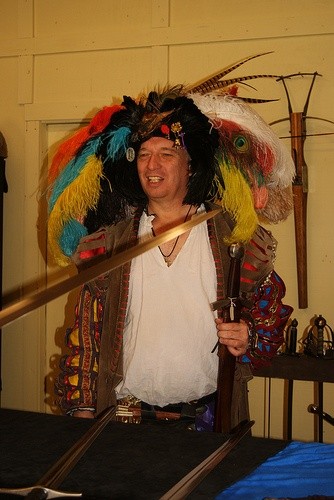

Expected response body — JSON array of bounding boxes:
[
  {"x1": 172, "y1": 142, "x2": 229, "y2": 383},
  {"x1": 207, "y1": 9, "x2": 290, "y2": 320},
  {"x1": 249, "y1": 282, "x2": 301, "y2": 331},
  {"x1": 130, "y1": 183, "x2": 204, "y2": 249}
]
[{"x1": 112, "y1": 408, "x2": 142, "y2": 424}]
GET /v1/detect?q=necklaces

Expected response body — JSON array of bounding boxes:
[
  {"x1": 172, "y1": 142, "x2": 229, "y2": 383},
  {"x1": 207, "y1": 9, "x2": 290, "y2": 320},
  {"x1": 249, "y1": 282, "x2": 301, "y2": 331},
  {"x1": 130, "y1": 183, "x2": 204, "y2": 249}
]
[{"x1": 146, "y1": 204, "x2": 192, "y2": 263}]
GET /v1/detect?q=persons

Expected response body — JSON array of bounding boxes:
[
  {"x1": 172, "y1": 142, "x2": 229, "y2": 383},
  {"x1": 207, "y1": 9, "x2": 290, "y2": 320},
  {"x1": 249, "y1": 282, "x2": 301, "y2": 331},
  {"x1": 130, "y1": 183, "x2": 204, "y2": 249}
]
[{"x1": 44, "y1": 84, "x2": 293, "y2": 434}]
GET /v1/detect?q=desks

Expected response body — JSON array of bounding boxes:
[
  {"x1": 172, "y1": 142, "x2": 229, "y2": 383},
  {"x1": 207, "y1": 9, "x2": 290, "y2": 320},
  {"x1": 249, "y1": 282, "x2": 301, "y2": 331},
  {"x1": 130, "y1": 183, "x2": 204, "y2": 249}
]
[{"x1": 0, "y1": 408, "x2": 334, "y2": 500}]
